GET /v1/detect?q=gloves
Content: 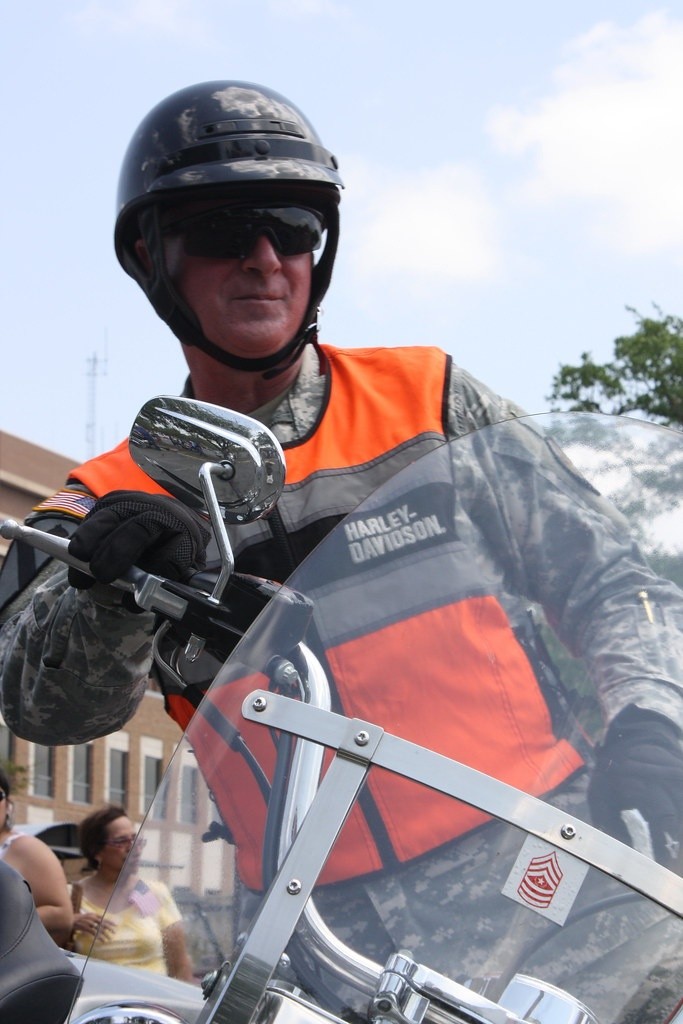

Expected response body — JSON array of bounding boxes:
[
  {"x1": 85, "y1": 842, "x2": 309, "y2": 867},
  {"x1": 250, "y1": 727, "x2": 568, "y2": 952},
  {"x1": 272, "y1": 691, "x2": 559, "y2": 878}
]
[
  {"x1": 586, "y1": 701, "x2": 682, "y2": 879},
  {"x1": 64, "y1": 488, "x2": 214, "y2": 616}
]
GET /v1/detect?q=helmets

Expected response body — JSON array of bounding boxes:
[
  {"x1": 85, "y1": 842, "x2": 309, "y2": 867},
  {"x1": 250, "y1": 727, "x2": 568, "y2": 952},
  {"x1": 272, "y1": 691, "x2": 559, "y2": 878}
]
[{"x1": 112, "y1": 79, "x2": 344, "y2": 278}]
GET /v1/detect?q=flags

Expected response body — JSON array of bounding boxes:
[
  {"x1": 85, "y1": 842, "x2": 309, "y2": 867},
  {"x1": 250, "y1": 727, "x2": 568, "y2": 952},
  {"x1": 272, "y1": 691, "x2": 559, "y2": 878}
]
[{"x1": 128, "y1": 879, "x2": 163, "y2": 918}]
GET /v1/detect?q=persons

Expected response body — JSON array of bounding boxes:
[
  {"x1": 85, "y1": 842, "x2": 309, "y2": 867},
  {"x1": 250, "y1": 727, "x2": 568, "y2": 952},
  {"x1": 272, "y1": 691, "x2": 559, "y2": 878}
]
[
  {"x1": 0, "y1": 767, "x2": 75, "y2": 947},
  {"x1": 0, "y1": 81, "x2": 683, "y2": 1024},
  {"x1": 67, "y1": 807, "x2": 193, "y2": 986}
]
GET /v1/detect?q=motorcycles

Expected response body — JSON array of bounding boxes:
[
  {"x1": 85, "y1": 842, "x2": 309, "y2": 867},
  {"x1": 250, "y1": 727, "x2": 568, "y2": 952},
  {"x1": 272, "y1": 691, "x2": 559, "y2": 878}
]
[{"x1": 0, "y1": 395, "x2": 683, "y2": 1024}]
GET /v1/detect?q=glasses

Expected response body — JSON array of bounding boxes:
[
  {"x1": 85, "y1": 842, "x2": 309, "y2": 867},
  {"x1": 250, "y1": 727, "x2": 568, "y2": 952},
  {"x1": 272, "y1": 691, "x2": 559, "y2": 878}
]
[
  {"x1": 104, "y1": 838, "x2": 148, "y2": 852},
  {"x1": 0, "y1": 788, "x2": 7, "y2": 800},
  {"x1": 176, "y1": 203, "x2": 324, "y2": 258}
]
